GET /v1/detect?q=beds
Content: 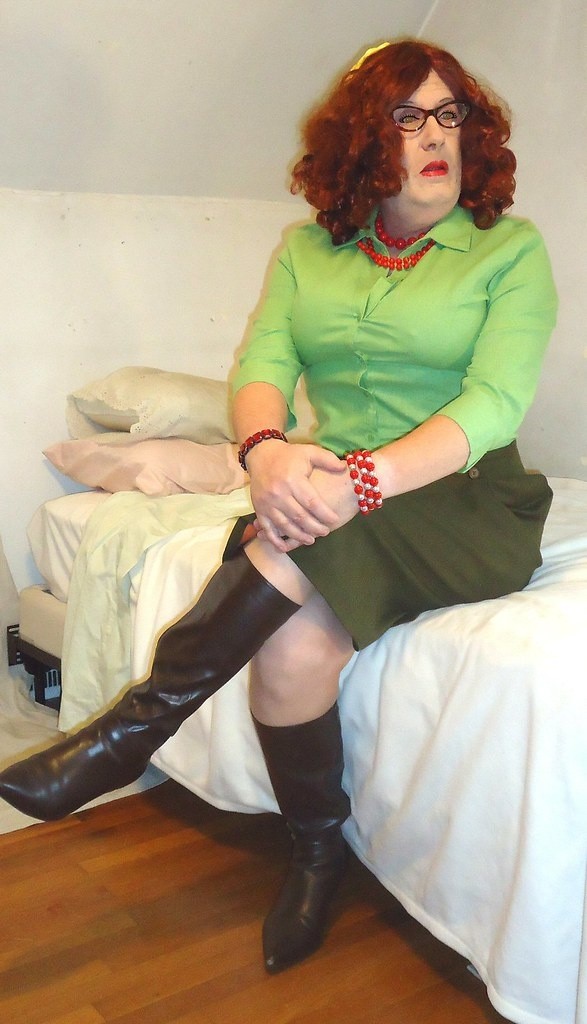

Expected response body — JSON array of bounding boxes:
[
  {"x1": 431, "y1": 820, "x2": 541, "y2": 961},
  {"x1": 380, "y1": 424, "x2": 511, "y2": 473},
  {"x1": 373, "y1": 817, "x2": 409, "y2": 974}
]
[{"x1": 18, "y1": 477, "x2": 587, "y2": 1024}]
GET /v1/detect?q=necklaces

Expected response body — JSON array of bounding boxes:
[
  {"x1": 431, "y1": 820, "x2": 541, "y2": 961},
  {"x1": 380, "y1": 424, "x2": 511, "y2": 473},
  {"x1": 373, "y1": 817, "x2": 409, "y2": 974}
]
[{"x1": 356, "y1": 210, "x2": 437, "y2": 271}]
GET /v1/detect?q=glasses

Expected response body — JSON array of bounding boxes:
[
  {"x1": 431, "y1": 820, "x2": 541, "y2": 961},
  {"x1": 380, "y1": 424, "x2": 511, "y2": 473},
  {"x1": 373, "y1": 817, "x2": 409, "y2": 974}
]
[{"x1": 391, "y1": 100, "x2": 470, "y2": 132}]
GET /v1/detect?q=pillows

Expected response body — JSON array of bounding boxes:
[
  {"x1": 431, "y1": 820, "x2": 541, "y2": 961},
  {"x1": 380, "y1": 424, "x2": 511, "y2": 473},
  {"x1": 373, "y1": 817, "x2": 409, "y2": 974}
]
[
  {"x1": 70, "y1": 366, "x2": 236, "y2": 442},
  {"x1": 49, "y1": 435, "x2": 250, "y2": 497}
]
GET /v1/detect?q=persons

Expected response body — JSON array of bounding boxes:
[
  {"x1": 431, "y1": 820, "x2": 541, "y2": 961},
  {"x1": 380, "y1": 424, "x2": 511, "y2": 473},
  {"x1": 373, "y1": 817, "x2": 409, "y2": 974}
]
[{"x1": 0, "y1": 41, "x2": 558, "y2": 976}]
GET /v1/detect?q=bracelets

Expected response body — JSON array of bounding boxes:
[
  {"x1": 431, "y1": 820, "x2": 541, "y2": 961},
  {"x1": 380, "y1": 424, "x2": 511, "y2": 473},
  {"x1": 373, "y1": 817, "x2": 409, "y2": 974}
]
[
  {"x1": 238, "y1": 428, "x2": 288, "y2": 473},
  {"x1": 345, "y1": 449, "x2": 382, "y2": 515}
]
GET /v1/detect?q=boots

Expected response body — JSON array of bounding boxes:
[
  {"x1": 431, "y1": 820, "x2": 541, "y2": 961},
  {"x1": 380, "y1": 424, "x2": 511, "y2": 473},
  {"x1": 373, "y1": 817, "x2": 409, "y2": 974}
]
[
  {"x1": 250, "y1": 700, "x2": 352, "y2": 976},
  {"x1": 0, "y1": 546, "x2": 303, "y2": 822}
]
